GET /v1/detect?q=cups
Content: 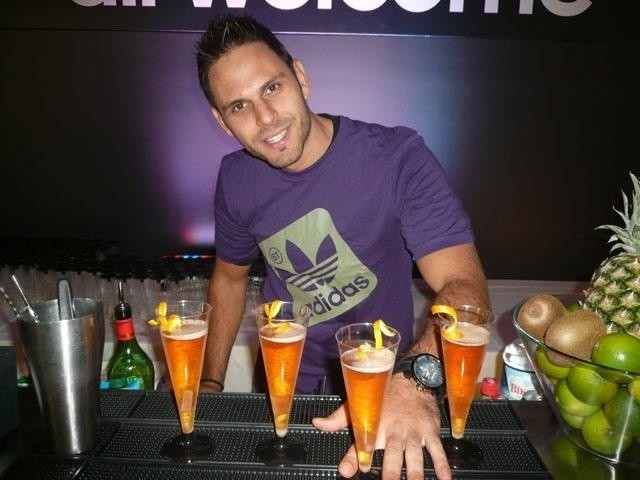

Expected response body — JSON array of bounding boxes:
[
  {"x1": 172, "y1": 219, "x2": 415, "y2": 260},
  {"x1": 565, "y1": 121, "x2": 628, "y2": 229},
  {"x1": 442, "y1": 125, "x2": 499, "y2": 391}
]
[{"x1": 16, "y1": 296, "x2": 106, "y2": 458}]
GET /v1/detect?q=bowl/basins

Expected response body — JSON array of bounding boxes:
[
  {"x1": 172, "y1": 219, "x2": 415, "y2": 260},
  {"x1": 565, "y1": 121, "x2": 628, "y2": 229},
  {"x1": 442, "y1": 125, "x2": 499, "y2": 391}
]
[{"x1": 510, "y1": 289, "x2": 640, "y2": 472}]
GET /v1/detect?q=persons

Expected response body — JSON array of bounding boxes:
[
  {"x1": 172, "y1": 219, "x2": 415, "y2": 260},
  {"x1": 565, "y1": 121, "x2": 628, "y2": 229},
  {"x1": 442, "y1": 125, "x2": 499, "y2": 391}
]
[{"x1": 159, "y1": 10, "x2": 494, "y2": 480}]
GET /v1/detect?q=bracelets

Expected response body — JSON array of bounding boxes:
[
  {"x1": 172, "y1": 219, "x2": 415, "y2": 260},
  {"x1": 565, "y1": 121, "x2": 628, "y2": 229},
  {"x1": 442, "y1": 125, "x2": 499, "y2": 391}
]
[{"x1": 197, "y1": 378, "x2": 226, "y2": 391}]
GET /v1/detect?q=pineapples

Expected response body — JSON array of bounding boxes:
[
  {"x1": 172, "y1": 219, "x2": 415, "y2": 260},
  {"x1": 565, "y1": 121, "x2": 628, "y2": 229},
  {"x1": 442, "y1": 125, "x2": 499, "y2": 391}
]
[{"x1": 578, "y1": 169, "x2": 640, "y2": 341}]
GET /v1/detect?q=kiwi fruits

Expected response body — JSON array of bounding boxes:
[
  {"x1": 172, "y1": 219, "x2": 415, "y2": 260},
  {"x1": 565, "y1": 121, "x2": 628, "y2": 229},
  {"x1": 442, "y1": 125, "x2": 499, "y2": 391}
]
[
  {"x1": 516, "y1": 294, "x2": 566, "y2": 339},
  {"x1": 543, "y1": 310, "x2": 607, "y2": 367}
]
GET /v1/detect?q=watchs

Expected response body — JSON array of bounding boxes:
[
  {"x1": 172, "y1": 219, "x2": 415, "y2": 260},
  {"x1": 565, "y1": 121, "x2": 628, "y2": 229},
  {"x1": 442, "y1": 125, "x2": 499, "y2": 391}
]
[{"x1": 389, "y1": 349, "x2": 448, "y2": 407}]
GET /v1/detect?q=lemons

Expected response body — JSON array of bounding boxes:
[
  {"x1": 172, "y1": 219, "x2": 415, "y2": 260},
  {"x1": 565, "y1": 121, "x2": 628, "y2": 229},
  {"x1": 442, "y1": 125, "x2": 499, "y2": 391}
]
[{"x1": 537, "y1": 334, "x2": 640, "y2": 459}]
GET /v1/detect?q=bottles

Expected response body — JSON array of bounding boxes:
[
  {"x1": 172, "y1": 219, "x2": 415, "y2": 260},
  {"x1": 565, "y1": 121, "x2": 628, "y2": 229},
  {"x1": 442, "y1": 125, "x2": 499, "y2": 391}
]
[{"x1": 104, "y1": 302, "x2": 155, "y2": 392}]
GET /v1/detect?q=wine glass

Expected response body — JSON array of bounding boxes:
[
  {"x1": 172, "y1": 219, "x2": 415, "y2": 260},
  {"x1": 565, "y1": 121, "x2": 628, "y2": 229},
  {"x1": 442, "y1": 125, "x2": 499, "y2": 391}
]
[
  {"x1": 427, "y1": 304, "x2": 498, "y2": 470},
  {"x1": 250, "y1": 300, "x2": 311, "y2": 469},
  {"x1": 334, "y1": 322, "x2": 403, "y2": 479},
  {"x1": 155, "y1": 299, "x2": 218, "y2": 462}
]
